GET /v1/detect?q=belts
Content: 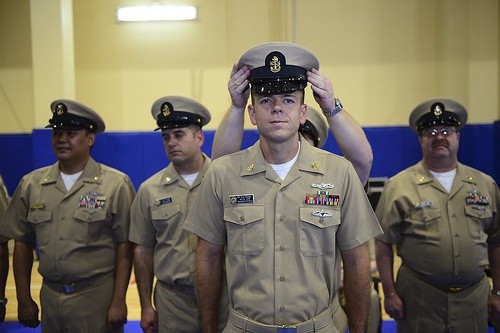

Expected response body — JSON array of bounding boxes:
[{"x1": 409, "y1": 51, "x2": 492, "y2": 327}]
[
  {"x1": 43, "y1": 271, "x2": 111, "y2": 295},
  {"x1": 229, "y1": 292, "x2": 340, "y2": 333},
  {"x1": 406, "y1": 269, "x2": 485, "y2": 294},
  {"x1": 157, "y1": 279, "x2": 194, "y2": 296}
]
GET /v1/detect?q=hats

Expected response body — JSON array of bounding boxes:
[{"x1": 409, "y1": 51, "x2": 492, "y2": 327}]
[
  {"x1": 299, "y1": 105, "x2": 329, "y2": 148},
  {"x1": 151, "y1": 95, "x2": 212, "y2": 132},
  {"x1": 408, "y1": 97, "x2": 469, "y2": 131},
  {"x1": 44, "y1": 99, "x2": 105, "y2": 135},
  {"x1": 235, "y1": 42, "x2": 320, "y2": 96}
]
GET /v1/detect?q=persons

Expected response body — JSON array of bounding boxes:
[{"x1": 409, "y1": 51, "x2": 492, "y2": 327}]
[
  {"x1": 182, "y1": 42, "x2": 383, "y2": 333},
  {"x1": 374, "y1": 99, "x2": 500, "y2": 333},
  {"x1": 127, "y1": 96, "x2": 230, "y2": 333},
  {"x1": 0, "y1": 99, "x2": 137, "y2": 333}
]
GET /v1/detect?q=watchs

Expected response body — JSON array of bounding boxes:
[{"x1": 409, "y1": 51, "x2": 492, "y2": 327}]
[{"x1": 322, "y1": 98, "x2": 343, "y2": 118}]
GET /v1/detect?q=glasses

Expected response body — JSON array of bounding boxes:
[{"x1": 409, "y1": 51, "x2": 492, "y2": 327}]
[{"x1": 417, "y1": 129, "x2": 460, "y2": 138}]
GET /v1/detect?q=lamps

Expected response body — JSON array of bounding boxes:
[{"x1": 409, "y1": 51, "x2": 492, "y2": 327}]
[{"x1": 115, "y1": 3, "x2": 197, "y2": 21}]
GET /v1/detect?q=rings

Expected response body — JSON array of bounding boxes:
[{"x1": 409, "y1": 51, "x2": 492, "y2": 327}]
[{"x1": 234, "y1": 82, "x2": 237, "y2": 88}]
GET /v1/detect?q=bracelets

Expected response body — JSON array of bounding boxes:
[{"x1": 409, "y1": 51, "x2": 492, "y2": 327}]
[{"x1": 491, "y1": 289, "x2": 500, "y2": 296}]
